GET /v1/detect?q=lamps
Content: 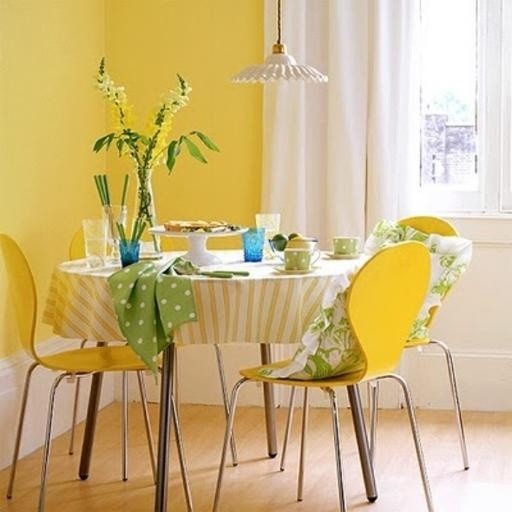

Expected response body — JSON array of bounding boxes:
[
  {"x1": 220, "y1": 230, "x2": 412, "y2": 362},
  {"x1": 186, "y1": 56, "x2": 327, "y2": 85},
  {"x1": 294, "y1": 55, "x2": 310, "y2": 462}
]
[{"x1": 230, "y1": 1, "x2": 329, "y2": 87}]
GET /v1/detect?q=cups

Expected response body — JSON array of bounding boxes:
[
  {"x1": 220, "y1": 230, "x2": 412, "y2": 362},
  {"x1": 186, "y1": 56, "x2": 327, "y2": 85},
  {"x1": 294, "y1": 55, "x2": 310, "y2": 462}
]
[
  {"x1": 119, "y1": 242, "x2": 139, "y2": 268},
  {"x1": 333, "y1": 237, "x2": 358, "y2": 256},
  {"x1": 282, "y1": 248, "x2": 319, "y2": 270},
  {"x1": 255, "y1": 212, "x2": 281, "y2": 236},
  {"x1": 241, "y1": 228, "x2": 265, "y2": 261},
  {"x1": 102, "y1": 206, "x2": 128, "y2": 268},
  {"x1": 82, "y1": 217, "x2": 107, "y2": 271}
]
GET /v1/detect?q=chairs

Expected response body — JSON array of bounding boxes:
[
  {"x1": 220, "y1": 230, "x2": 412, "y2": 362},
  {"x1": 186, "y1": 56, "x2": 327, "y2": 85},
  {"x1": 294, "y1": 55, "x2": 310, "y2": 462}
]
[
  {"x1": 279, "y1": 217, "x2": 470, "y2": 472},
  {"x1": 70, "y1": 220, "x2": 246, "y2": 484},
  {"x1": 214, "y1": 241, "x2": 433, "y2": 512},
  {"x1": 1, "y1": 234, "x2": 194, "y2": 512}
]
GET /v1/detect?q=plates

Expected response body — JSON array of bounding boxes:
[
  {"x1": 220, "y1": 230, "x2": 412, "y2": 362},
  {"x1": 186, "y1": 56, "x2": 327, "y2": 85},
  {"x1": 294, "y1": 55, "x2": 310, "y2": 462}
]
[
  {"x1": 276, "y1": 266, "x2": 319, "y2": 274},
  {"x1": 325, "y1": 252, "x2": 359, "y2": 259},
  {"x1": 146, "y1": 220, "x2": 249, "y2": 266}
]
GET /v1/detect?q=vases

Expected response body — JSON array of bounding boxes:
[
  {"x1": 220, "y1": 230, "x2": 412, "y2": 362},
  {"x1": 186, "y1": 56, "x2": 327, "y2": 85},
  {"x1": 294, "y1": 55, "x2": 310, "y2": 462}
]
[{"x1": 134, "y1": 168, "x2": 161, "y2": 264}]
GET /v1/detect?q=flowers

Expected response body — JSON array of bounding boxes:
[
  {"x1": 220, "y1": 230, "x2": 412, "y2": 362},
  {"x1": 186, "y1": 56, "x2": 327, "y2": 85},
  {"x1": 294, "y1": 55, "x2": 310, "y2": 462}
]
[{"x1": 92, "y1": 56, "x2": 219, "y2": 255}]
